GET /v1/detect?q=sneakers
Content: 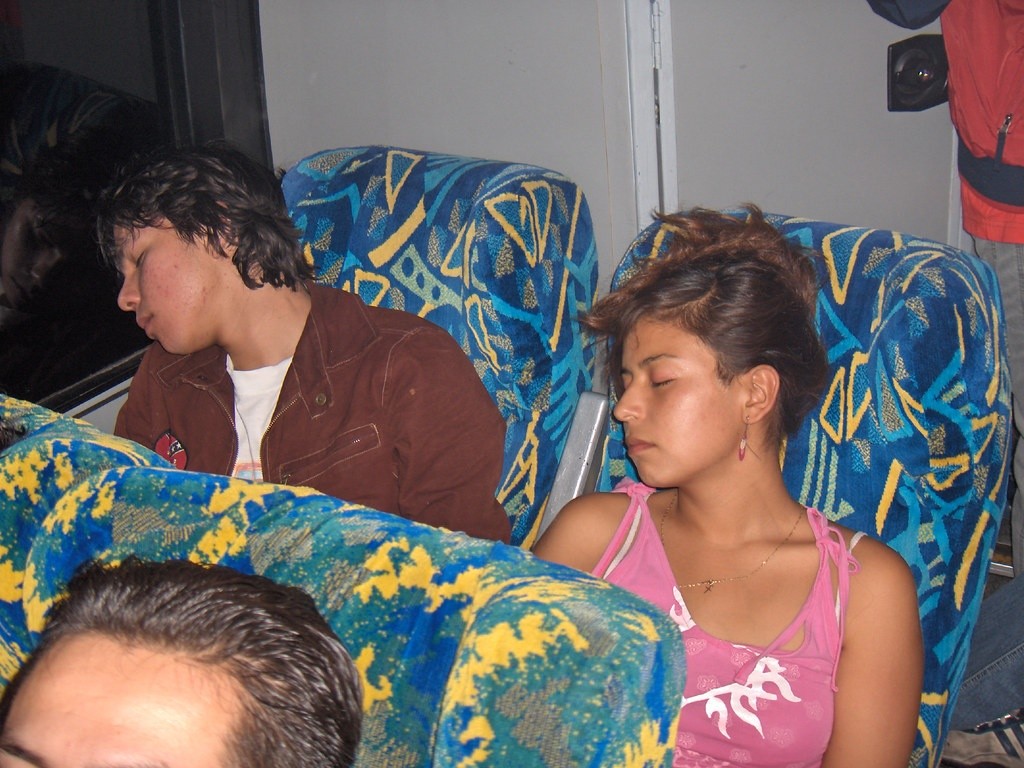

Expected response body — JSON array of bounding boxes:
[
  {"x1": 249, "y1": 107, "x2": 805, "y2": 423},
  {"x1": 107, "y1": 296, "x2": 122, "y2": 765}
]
[{"x1": 941, "y1": 709, "x2": 1024, "y2": 768}]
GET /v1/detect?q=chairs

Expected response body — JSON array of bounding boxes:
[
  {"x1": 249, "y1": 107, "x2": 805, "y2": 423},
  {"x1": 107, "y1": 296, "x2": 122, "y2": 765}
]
[
  {"x1": 280, "y1": 144, "x2": 601, "y2": 540},
  {"x1": 0, "y1": 393, "x2": 172, "y2": 694},
  {"x1": 23, "y1": 466, "x2": 692, "y2": 768},
  {"x1": 546, "y1": 210, "x2": 1013, "y2": 768}
]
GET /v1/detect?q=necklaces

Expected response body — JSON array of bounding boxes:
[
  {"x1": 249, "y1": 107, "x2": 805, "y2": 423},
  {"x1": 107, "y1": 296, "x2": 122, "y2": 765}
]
[
  {"x1": 236, "y1": 408, "x2": 255, "y2": 476},
  {"x1": 660, "y1": 494, "x2": 804, "y2": 593}
]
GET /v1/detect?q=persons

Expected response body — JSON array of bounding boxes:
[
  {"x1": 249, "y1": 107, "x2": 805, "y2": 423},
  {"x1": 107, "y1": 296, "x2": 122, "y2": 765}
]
[
  {"x1": 865, "y1": 0, "x2": 1024, "y2": 768},
  {"x1": 532, "y1": 207, "x2": 924, "y2": 768},
  {"x1": 0, "y1": 557, "x2": 365, "y2": 768},
  {"x1": 95, "y1": 136, "x2": 510, "y2": 549}
]
